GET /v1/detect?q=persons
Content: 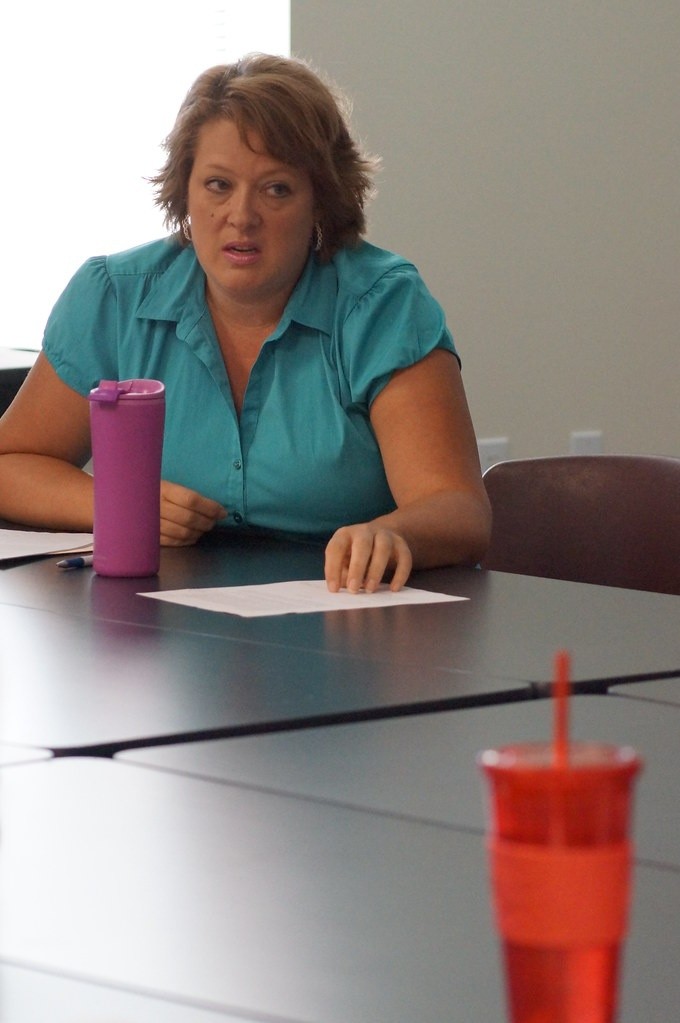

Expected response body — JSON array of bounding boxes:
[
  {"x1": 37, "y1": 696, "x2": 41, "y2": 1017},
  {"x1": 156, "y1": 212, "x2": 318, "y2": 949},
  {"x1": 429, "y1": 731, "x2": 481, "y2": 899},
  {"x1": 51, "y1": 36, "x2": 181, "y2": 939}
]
[{"x1": 0, "y1": 52, "x2": 494, "y2": 593}]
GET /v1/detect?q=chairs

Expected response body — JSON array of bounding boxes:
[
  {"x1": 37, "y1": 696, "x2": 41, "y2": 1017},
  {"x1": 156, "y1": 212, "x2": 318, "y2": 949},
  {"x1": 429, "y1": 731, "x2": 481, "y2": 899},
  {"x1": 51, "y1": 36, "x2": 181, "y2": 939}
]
[{"x1": 479, "y1": 453, "x2": 680, "y2": 595}]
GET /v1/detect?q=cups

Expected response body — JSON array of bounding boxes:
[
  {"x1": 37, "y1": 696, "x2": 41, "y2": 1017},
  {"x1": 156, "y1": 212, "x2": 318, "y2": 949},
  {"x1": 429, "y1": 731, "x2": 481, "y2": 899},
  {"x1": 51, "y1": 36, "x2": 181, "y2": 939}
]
[{"x1": 476, "y1": 742, "x2": 646, "y2": 1022}]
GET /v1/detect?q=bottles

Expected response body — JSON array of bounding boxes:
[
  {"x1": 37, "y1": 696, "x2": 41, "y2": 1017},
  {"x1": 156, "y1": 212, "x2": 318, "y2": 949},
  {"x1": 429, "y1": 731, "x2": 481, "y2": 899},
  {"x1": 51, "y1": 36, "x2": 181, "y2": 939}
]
[{"x1": 88, "y1": 377, "x2": 164, "y2": 577}]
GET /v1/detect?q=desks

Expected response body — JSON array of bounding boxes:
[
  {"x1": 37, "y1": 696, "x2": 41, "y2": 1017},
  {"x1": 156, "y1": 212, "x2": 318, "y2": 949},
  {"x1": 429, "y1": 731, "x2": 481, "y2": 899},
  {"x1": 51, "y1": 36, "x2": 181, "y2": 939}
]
[{"x1": 0, "y1": 524, "x2": 680, "y2": 1023}]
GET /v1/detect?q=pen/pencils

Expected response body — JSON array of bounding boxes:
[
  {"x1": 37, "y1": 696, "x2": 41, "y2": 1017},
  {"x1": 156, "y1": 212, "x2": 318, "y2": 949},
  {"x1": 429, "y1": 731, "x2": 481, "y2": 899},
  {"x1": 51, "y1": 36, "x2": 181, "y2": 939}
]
[{"x1": 56, "y1": 555, "x2": 93, "y2": 568}]
